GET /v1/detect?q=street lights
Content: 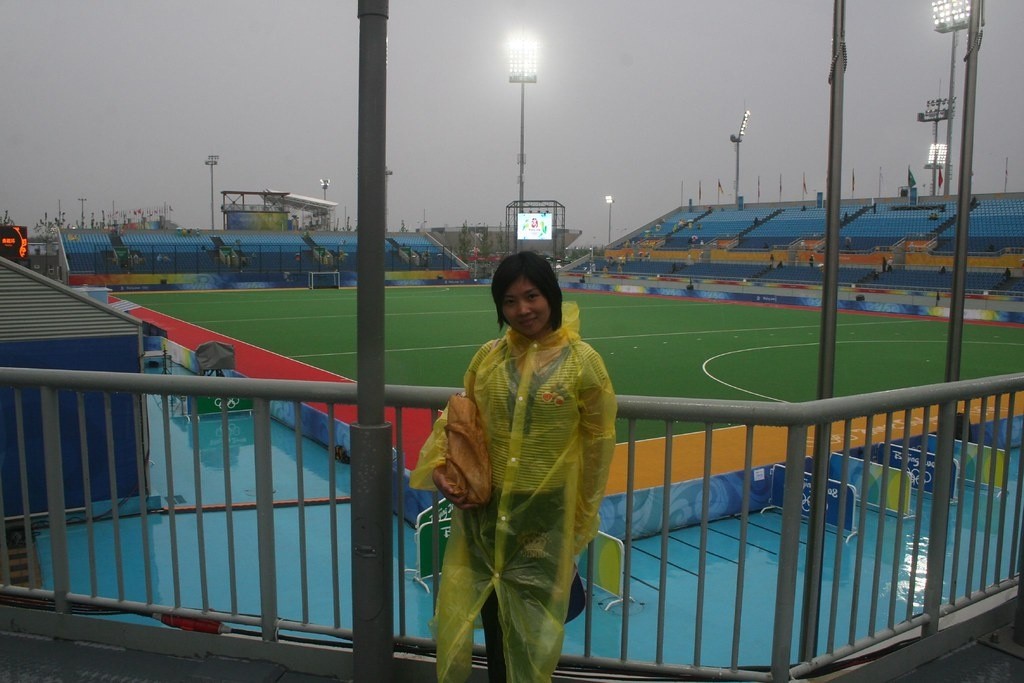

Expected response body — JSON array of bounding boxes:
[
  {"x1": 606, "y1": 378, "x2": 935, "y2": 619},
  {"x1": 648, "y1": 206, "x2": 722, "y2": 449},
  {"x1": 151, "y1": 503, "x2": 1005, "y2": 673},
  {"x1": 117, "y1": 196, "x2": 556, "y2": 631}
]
[
  {"x1": 932, "y1": 0, "x2": 971, "y2": 196},
  {"x1": 926, "y1": 143, "x2": 948, "y2": 195},
  {"x1": 507, "y1": 38, "x2": 539, "y2": 255},
  {"x1": 734, "y1": 110, "x2": 751, "y2": 205}
]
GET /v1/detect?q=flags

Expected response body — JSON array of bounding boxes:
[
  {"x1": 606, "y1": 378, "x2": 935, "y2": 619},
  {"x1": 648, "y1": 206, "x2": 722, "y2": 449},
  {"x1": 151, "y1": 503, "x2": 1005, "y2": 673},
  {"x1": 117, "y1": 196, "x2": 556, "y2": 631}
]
[
  {"x1": 909, "y1": 166, "x2": 943, "y2": 188},
  {"x1": 718, "y1": 174, "x2": 856, "y2": 194},
  {"x1": 116, "y1": 208, "x2": 173, "y2": 215}
]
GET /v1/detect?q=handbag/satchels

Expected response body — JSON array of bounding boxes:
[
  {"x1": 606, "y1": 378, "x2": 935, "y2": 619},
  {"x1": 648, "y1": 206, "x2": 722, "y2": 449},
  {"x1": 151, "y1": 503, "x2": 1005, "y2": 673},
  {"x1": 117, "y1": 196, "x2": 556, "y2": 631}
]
[{"x1": 444, "y1": 394, "x2": 493, "y2": 509}]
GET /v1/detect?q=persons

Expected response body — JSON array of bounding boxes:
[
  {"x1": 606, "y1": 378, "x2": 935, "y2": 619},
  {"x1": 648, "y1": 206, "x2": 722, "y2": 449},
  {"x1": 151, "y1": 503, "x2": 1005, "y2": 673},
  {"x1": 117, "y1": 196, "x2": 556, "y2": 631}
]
[
  {"x1": 603, "y1": 219, "x2": 704, "y2": 273},
  {"x1": 410, "y1": 249, "x2": 615, "y2": 683},
  {"x1": 754, "y1": 206, "x2": 1010, "y2": 302}
]
